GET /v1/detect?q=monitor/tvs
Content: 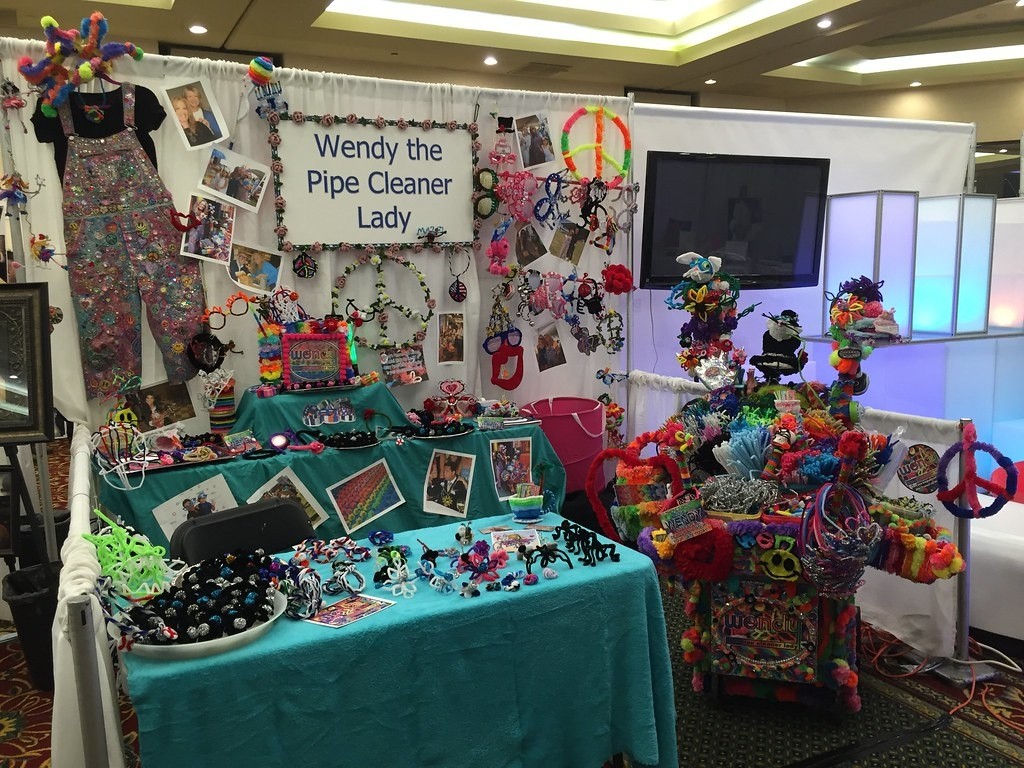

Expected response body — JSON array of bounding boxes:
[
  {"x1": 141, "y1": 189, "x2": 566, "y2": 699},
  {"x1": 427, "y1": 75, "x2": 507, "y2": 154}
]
[{"x1": 638, "y1": 151, "x2": 831, "y2": 291}]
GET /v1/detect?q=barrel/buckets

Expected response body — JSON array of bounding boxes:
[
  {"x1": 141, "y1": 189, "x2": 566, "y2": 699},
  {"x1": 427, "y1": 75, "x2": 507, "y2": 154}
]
[{"x1": 520, "y1": 397, "x2": 606, "y2": 492}]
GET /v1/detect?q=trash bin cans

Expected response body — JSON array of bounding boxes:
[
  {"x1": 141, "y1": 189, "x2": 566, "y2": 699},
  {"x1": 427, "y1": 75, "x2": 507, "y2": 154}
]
[
  {"x1": 18, "y1": 507, "x2": 71, "y2": 562},
  {"x1": 1, "y1": 559, "x2": 65, "y2": 691}
]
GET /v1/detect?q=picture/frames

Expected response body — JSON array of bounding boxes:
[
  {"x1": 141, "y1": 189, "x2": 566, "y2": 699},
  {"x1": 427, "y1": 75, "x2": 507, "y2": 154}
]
[{"x1": 0, "y1": 281, "x2": 56, "y2": 446}]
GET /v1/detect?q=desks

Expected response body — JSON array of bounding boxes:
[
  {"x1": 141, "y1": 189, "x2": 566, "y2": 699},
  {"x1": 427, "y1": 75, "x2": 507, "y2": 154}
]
[{"x1": 116, "y1": 502, "x2": 681, "y2": 768}]
